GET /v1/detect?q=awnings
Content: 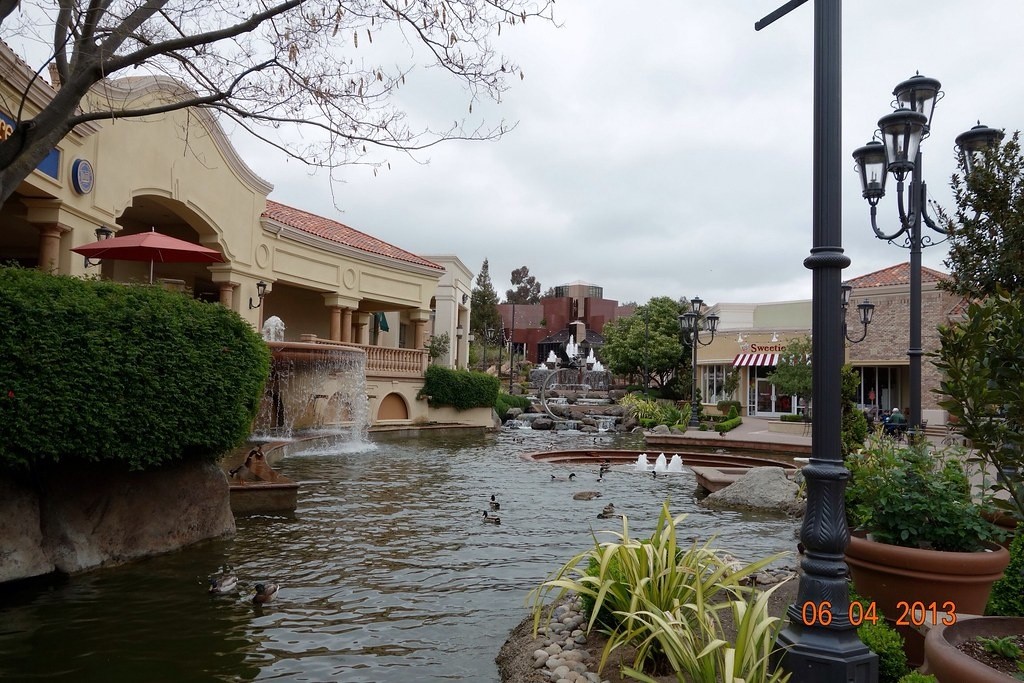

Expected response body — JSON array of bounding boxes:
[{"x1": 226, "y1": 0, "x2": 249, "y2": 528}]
[{"x1": 731, "y1": 353, "x2": 812, "y2": 368}]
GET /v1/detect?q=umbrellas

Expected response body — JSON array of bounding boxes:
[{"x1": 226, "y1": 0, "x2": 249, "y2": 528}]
[{"x1": 69, "y1": 227, "x2": 227, "y2": 288}]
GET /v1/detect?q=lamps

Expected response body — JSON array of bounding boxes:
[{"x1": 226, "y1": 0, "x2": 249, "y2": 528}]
[
  {"x1": 249, "y1": 280, "x2": 267, "y2": 310},
  {"x1": 84, "y1": 221, "x2": 115, "y2": 269}
]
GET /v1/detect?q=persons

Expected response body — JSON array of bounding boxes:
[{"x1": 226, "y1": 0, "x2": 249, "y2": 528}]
[{"x1": 861, "y1": 405, "x2": 907, "y2": 440}]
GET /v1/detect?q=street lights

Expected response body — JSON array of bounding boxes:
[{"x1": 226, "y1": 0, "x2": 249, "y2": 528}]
[
  {"x1": 841, "y1": 280, "x2": 875, "y2": 434},
  {"x1": 482, "y1": 323, "x2": 495, "y2": 372},
  {"x1": 849, "y1": 69, "x2": 1003, "y2": 450},
  {"x1": 678, "y1": 295, "x2": 720, "y2": 427}
]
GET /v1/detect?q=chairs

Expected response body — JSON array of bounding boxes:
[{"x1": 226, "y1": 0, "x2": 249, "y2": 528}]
[{"x1": 801, "y1": 405, "x2": 929, "y2": 445}]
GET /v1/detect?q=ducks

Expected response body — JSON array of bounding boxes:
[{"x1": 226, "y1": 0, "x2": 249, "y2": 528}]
[
  {"x1": 248, "y1": 583, "x2": 280, "y2": 604},
  {"x1": 650, "y1": 470, "x2": 668, "y2": 479},
  {"x1": 545, "y1": 442, "x2": 556, "y2": 451},
  {"x1": 593, "y1": 437, "x2": 605, "y2": 445},
  {"x1": 513, "y1": 437, "x2": 525, "y2": 444},
  {"x1": 482, "y1": 494, "x2": 500, "y2": 525},
  {"x1": 550, "y1": 472, "x2": 577, "y2": 483},
  {"x1": 209, "y1": 574, "x2": 238, "y2": 593},
  {"x1": 228, "y1": 449, "x2": 261, "y2": 487},
  {"x1": 596, "y1": 460, "x2": 612, "y2": 484},
  {"x1": 603, "y1": 502, "x2": 615, "y2": 514}
]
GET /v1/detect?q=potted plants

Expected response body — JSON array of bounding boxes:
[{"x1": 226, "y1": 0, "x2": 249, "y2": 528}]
[
  {"x1": 842, "y1": 424, "x2": 1012, "y2": 672},
  {"x1": 716, "y1": 365, "x2": 742, "y2": 416},
  {"x1": 922, "y1": 614, "x2": 1023, "y2": 683}
]
